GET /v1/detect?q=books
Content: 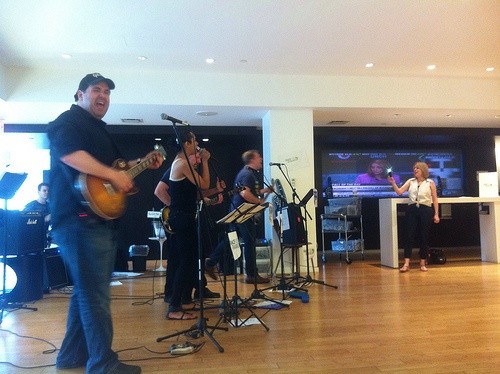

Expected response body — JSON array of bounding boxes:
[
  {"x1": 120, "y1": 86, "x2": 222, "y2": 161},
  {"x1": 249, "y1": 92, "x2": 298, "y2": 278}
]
[{"x1": 217, "y1": 203, "x2": 264, "y2": 223}]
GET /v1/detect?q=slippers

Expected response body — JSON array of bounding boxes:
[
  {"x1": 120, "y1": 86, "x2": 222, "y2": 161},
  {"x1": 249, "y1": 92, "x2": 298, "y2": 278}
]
[
  {"x1": 182, "y1": 304, "x2": 200, "y2": 310},
  {"x1": 168, "y1": 311, "x2": 196, "y2": 319}
]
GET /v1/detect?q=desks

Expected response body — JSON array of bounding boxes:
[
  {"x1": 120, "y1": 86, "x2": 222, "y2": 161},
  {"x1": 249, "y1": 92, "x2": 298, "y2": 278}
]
[
  {"x1": 148, "y1": 237, "x2": 168, "y2": 272},
  {"x1": 379, "y1": 197, "x2": 500, "y2": 269}
]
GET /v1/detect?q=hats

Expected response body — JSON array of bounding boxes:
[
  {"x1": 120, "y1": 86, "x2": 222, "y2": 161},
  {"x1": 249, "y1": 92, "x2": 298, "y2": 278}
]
[{"x1": 79, "y1": 73, "x2": 115, "y2": 89}]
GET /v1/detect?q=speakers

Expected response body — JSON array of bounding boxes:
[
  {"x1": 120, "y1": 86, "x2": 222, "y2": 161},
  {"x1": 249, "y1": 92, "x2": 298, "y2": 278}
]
[
  {"x1": 0, "y1": 209, "x2": 75, "y2": 303},
  {"x1": 275, "y1": 202, "x2": 307, "y2": 244}
]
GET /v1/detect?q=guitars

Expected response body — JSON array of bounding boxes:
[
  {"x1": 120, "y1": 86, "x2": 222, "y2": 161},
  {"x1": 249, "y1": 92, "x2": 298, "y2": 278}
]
[
  {"x1": 250, "y1": 178, "x2": 275, "y2": 226},
  {"x1": 160, "y1": 183, "x2": 246, "y2": 234},
  {"x1": 79, "y1": 138, "x2": 167, "y2": 220}
]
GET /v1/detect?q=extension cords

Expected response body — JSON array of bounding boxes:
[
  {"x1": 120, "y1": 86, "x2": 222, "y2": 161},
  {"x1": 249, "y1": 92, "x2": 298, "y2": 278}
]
[{"x1": 170, "y1": 343, "x2": 194, "y2": 355}]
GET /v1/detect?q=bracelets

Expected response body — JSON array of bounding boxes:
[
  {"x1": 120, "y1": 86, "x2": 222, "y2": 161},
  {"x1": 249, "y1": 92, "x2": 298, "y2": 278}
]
[{"x1": 137, "y1": 160, "x2": 141, "y2": 164}]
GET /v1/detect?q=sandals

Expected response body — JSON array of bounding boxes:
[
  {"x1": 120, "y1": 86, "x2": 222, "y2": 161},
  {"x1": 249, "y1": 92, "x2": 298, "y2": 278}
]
[
  {"x1": 400, "y1": 266, "x2": 409, "y2": 271},
  {"x1": 420, "y1": 264, "x2": 428, "y2": 271}
]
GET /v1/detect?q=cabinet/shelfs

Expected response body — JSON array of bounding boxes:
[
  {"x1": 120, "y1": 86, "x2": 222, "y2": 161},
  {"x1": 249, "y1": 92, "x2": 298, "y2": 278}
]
[{"x1": 320, "y1": 213, "x2": 366, "y2": 264}]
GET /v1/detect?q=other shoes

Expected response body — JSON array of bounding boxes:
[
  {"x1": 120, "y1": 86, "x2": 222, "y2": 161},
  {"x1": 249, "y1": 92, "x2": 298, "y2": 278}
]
[
  {"x1": 246, "y1": 276, "x2": 269, "y2": 283},
  {"x1": 109, "y1": 363, "x2": 141, "y2": 374},
  {"x1": 205, "y1": 264, "x2": 218, "y2": 280},
  {"x1": 194, "y1": 288, "x2": 220, "y2": 297}
]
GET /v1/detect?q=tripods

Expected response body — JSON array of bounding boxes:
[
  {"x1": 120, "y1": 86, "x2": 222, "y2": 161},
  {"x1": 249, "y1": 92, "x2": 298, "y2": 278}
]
[
  {"x1": 156, "y1": 123, "x2": 230, "y2": 353},
  {"x1": 244, "y1": 166, "x2": 260, "y2": 173},
  {"x1": 268, "y1": 162, "x2": 285, "y2": 166},
  {"x1": 200, "y1": 157, "x2": 340, "y2": 336}
]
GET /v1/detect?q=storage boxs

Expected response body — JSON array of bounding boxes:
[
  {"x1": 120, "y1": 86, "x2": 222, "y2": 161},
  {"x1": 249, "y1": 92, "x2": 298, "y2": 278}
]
[{"x1": 332, "y1": 239, "x2": 363, "y2": 252}]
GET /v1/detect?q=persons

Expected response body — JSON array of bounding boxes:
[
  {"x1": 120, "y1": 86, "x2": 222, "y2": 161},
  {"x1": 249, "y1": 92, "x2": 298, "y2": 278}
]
[
  {"x1": 163, "y1": 132, "x2": 211, "y2": 320},
  {"x1": 389, "y1": 162, "x2": 439, "y2": 271},
  {"x1": 356, "y1": 158, "x2": 400, "y2": 185},
  {"x1": 205, "y1": 150, "x2": 274, "y2": 283},
  {"x1": 48, "y1": 73, "x2": 160, "y2": 374},
  {"x1": 24, "y1": 183, "x2": 55, "y2": 294},
  {"x1": 154, "y1": 151, "x2": 223, "y2": 298}
]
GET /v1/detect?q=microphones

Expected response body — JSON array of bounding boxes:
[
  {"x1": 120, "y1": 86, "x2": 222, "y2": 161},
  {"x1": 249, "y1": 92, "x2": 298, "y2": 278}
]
[
  {"x1": 388, "y1": 169, "x2": 392, "y2": 179},
  {"x1": 160, "y1": 112, "x2": 188, "y2": 125},
  {"x1": 197, "y1": 146, "x2": 216, "y2": 161}
]
